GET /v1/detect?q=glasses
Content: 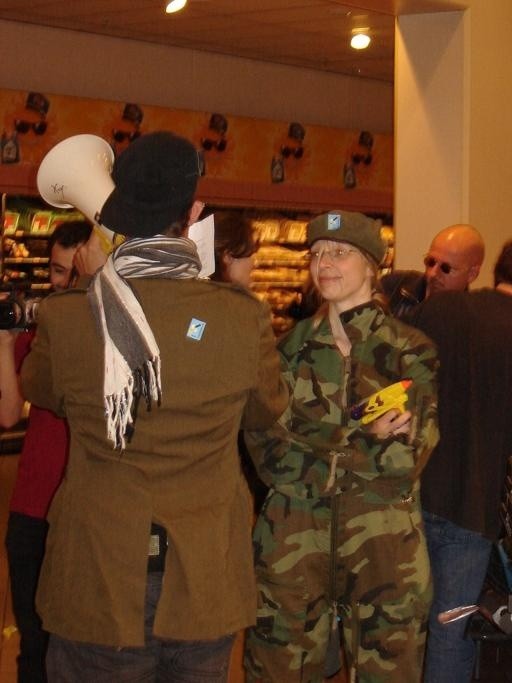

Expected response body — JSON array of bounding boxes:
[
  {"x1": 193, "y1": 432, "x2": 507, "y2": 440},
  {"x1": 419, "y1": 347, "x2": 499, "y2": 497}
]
[
  {"x1": 310, "y1": 248, "x2": 356, "y2": 260},
  {"x1": 423, "y1": 256, "x2": 463, "y2": 275}
]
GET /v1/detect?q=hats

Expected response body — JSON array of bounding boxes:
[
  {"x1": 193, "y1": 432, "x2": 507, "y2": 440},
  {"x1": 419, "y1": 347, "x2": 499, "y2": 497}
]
[
  {"x1": 99, "y1": 130, "x2": 199, "y2": 239},
  {"x1": 306, "y1": 209, "x2": 386, "y2": 266}
]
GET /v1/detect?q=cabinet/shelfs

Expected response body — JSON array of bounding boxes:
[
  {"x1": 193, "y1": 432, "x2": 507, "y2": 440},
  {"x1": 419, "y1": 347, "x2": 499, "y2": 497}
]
[
  {"x1": 2, "y1": 199, "x2": 84, "y2": 295},
  {"x1": 229, "y1": 211, "x2": 313, "y2": 332}
]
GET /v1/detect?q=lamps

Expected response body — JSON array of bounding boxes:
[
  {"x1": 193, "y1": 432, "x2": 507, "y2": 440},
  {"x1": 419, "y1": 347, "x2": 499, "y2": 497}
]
[
  {"x1": 12, "y1": 86, "x2": 53, "y2": 134},
  {"x1": 199, "y1": 113, "x2": 230, "y2": 150},
  {"x1": 347, "y1": 129, "x2": 378, "y2": 162},
  {"x1": 276, "y1": 119, "x2": 311, "y2": 160},
  {"x1": 108, "y1": 98, "x2": 148, "y2": 142}
]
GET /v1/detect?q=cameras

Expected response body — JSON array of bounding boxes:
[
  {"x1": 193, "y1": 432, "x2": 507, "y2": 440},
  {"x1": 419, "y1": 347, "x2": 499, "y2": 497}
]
[{"x1": 0, "y1": 293, "x2": 41, "y2": 330}]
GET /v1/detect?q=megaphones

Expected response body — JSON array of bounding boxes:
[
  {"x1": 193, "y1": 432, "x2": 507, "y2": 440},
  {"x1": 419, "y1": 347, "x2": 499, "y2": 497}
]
[{"x1": 36, "y1": 134, "x2": 129, "y2": 257}]
